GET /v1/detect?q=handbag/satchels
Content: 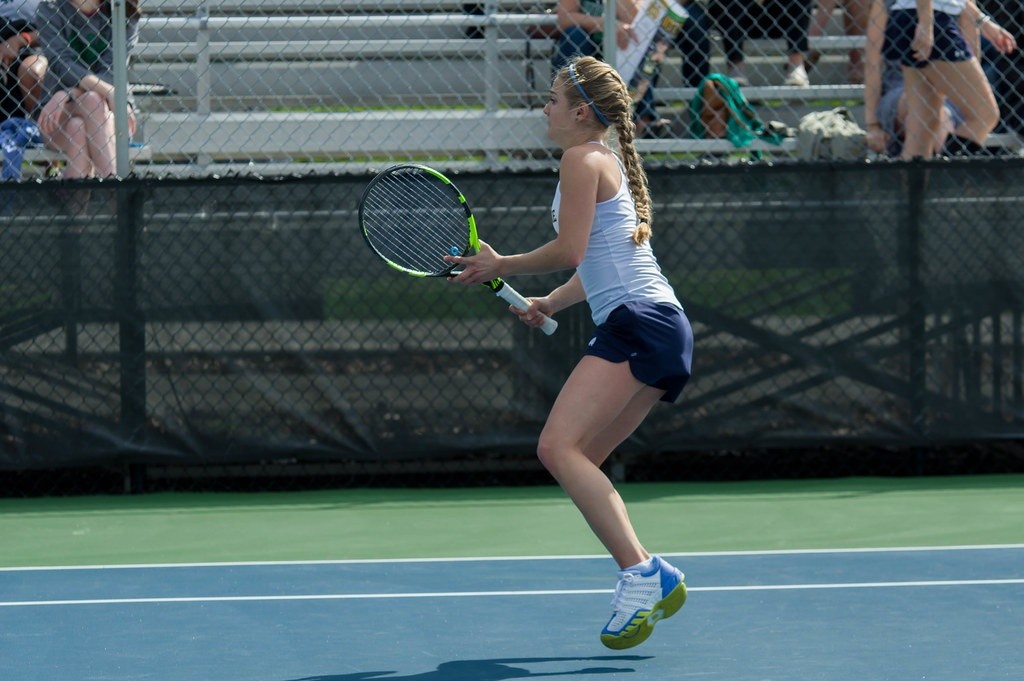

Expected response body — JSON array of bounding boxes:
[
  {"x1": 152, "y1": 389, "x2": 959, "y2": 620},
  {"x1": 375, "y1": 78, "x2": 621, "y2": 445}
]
[{"x1": 796, "y1": 106, "x2": 868, "y2": 161}]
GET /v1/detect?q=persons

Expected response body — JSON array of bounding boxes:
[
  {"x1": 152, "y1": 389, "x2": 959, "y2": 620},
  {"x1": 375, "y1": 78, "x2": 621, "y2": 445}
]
[
  {"x1": 550, "y1": 1, "x2": 673, "y2": 140},
  {"x1": 0, "y1": 0, "x2": 48, "y2": 122},
  {"x1": 632, "y1": 37, "x2": 670, "y2": 103},
  {"x1": 442, "y1": 56, "x2": 693, "y2": 651},
  {"x1": 670, "y1": 0, "x2": 1024, "y2": 160},
  {"x1": 33, "y1": 0, "x2": 142, "y2": 231}
]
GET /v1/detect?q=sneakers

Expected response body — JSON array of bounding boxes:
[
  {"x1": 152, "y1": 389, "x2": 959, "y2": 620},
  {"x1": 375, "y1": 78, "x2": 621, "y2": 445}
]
[{"x1": 600, "y1": 555, "x2": 687, "y2": 650}]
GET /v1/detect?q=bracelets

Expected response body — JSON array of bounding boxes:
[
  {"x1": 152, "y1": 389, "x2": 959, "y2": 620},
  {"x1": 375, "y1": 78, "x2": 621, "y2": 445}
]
[
  {"x1": 974, "y1": 13, "x2": 990, "y2": 27},
  {"x1": 21, "y1": 32, "x2": 30, "y2": 48},
  {"x1": 127, "y1": 101, "x2": 134, "y2": 109},
  {"x1": 864, "y1": 121, "x2": 882, "y2": 129},
  {"x1": 52, "y1": 87, "x2": 72, "y2": 103}
]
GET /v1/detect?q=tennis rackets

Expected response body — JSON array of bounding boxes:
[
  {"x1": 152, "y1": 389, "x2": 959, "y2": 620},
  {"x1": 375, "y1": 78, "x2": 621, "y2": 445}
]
[
  {"x1": 357, "y1": 164, "x2": 559, "y2": 336},
  {"x1": 129, "y1": 82, "x2": 174, "y2": 96}
]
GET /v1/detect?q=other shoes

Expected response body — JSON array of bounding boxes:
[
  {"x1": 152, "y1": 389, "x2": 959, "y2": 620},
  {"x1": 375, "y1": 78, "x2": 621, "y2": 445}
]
[
  {"x1": 729, "y1": 66, "x2": 749, "y2": 87},
  {"x1": 636, "y1": 118, "x2": 672, "y2": 139},
  {"x1": 784, "y1": 61, "x2": 810, "y2": 86}
]
